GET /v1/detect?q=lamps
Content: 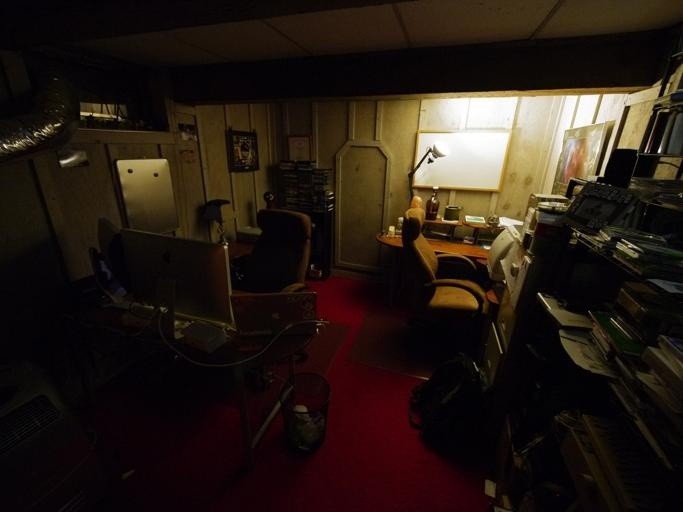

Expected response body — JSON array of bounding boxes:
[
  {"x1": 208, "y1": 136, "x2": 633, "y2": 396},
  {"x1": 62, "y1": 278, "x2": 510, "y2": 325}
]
[{"x1": 408, "y1": 144, "x2": 447, "y2": 199}]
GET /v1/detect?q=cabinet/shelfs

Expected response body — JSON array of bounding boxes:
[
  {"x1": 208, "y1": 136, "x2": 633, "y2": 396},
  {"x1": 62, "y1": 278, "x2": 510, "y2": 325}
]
[{"x1": 280, "y1": 207, "x2": 334, "y2": 279}]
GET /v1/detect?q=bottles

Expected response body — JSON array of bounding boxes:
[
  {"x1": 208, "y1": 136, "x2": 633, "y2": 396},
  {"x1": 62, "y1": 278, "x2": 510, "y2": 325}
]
[{"x1": 426, "y1": 189, "x2": 440, "y2": 221}]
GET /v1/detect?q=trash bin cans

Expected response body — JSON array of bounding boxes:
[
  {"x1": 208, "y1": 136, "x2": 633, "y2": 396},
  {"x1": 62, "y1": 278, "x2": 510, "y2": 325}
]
[{"x1": 277, "y1": 372, "x2": 331, "y2": 456}]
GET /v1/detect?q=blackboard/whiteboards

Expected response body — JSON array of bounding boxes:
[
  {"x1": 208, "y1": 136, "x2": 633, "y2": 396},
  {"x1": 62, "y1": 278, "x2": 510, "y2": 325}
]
[{"x1": 411, "y1": 129, "x2": 512, "y2": 193}]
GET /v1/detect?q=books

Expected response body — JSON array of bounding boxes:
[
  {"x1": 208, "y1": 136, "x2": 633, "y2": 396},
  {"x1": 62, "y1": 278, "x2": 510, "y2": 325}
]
[{"x1": 573, "y1": 219, "x2": 683, "y2": 469}]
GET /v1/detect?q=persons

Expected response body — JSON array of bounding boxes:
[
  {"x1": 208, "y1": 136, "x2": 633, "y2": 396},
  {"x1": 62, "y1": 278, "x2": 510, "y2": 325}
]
[{"x1": 404, "y1": 195, "x2": 425, "y2": 231}]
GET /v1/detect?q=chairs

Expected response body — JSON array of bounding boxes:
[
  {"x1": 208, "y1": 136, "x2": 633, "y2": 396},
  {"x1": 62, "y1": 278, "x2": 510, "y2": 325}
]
[
  {"x1": 402, "y1": 208, "x2": 489, "y2": 347},
  {"x1": 237, "y1": 209, "x2": 312, "y2": 292}
]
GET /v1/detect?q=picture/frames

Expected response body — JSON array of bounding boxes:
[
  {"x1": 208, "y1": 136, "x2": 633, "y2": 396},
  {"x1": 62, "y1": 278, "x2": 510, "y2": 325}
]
[{"x1": 287, "y1": 136, "x2": 311, "y2": 162}]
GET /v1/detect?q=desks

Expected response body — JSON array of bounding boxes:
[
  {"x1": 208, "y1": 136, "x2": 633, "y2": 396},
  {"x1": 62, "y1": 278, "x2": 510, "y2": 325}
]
[
  {"x1": 376, "y1": 231, "x2": 491, "y2": 308},
  {"x1": 48, "y1": 242, "x2": 317, "y2": 476}
]
